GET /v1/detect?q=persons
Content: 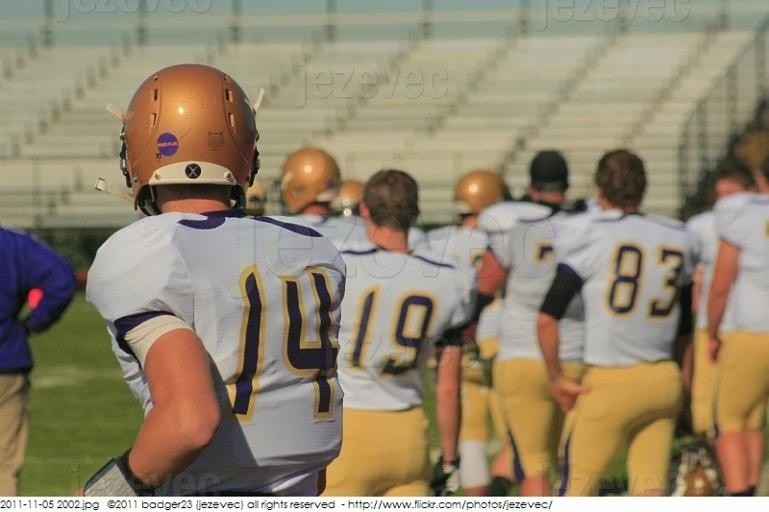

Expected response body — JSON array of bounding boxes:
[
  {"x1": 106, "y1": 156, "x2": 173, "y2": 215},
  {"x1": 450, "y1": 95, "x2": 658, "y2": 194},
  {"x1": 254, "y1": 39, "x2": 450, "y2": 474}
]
[
  {"x1": 429, "y1": 170, "x2": 522, "y2": 496},
  {"x1": 322, "y1": 168, "x2": 469, "y2": 497},
  {"x1": 333, "y1": 176, "x2": 371, "y2": 216},
  {"x1": 0, "y1": 227, "x2": 79, "y2": 496},
  {"x1": 689, "y1": 166, "x2": 763, "y2": 495},
  {"x1": 244, "y1": 179, "x2": 270, "y2": 209},
  {"x1": 81, "y1": 64, "x2": 346, "y2": 499},
  {"x1": 470, "y1": 151, "x2": 586, "y2": 496},
  {"x1": 538, "y1": 151, "x2": 699, "y2": 495},
  {"x1": 708, "y1": 130, "x2": 769, "y2": 496},
  {"x1": 268, "y1": 147, "x2": 425, "y2": 257}
]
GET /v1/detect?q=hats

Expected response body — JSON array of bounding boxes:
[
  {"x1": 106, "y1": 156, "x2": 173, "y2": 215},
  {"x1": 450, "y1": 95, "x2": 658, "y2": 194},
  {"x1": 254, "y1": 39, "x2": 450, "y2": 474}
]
[{"x1": 529, "y1": 151, "x2": 568, "y2": 183}]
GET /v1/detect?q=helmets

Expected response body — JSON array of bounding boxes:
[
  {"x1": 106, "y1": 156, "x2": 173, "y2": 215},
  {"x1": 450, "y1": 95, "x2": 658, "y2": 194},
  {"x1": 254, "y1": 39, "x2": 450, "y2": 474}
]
[
  {"x1": 329, "y1": 181, "x2": 365, "y2": 213},
  {"x1": 121, "y1": 64, "x2": 260, "y2": 211},
  {"x1": 278, "y1": 147, "x2": 342, "y2": 214},
  {"x1": 243, "y1": 178, "x2": 267, "y2": 216},
  {"x1": 453, "y1": 172, "x2": 507, "y2": 214}
]
